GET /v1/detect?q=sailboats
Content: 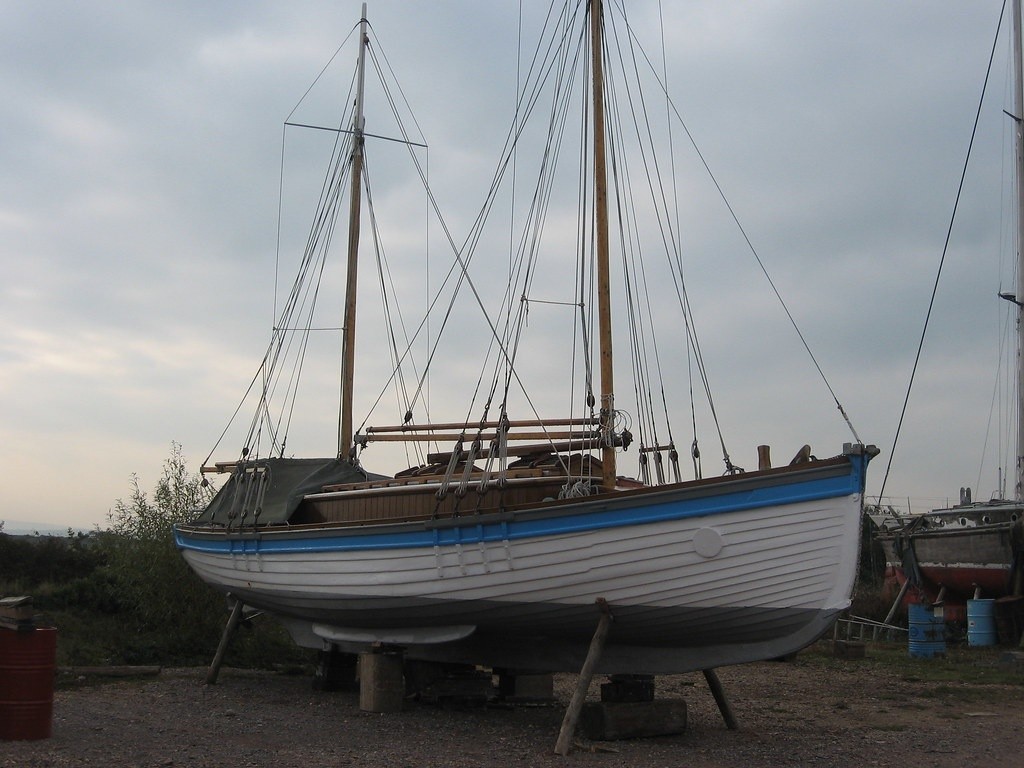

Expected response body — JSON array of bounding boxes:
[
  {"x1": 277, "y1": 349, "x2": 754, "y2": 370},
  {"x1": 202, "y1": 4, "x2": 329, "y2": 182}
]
[{"x1": 173, "y1": 0, "x2": 1024, "y2": 676}]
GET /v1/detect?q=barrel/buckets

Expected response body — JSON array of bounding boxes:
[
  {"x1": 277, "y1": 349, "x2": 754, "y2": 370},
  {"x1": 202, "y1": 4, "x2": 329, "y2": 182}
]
[
  {"x1": 966, "y1": 598, "x2": 996, "y2": 648},
  {"x1": 994, "y1": 595, "x2": 1024, "y2": 647},
  {"x1": 908, "y1": 602, "x2": 946, "y2": 660},
  {"x1": 0, "y1": 624, "x2": 58, "y2": 740}
]
